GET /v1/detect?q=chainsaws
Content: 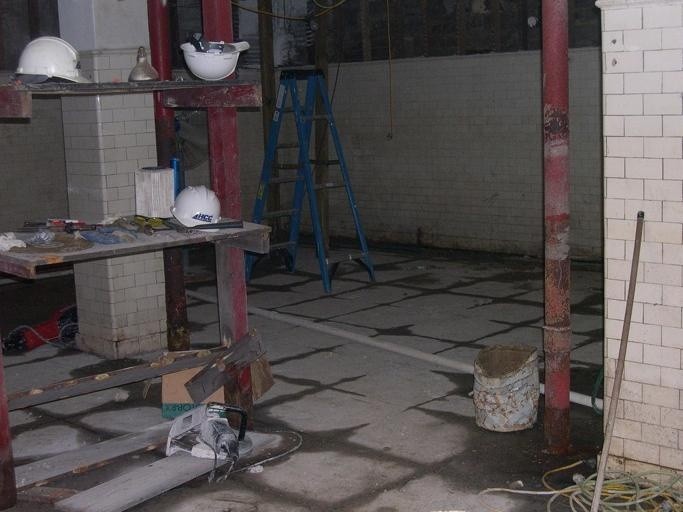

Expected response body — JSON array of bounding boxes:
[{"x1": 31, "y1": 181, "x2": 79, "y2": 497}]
[{"x1": 164, "y1": 401, "x2": 254, "y2": 460}]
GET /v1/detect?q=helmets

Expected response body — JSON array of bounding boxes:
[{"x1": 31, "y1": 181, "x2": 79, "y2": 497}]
[
  {"x1": 170, "y1": 184, "x2": 222, "y2": 233},
  {"x1": 180, "y1": 40, "x2": 250, "y2": 81},
  {"x1": 15, "y1": 36, "x2": 97, "y2": 83}
]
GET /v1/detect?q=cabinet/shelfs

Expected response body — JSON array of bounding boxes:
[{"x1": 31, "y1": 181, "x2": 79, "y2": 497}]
[{"x1": 1, "y1": 70, "x2": 274, "y2": 511}]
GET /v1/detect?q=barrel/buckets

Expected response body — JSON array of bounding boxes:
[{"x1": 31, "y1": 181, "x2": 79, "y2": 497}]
[{"x1": 467, "y1": 344, "x2": 540, "y2": 433}]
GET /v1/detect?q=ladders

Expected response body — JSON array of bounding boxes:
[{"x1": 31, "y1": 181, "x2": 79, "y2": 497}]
[{"x1": 244, "y1": 68, "x2": 377, "y2": 293}]
